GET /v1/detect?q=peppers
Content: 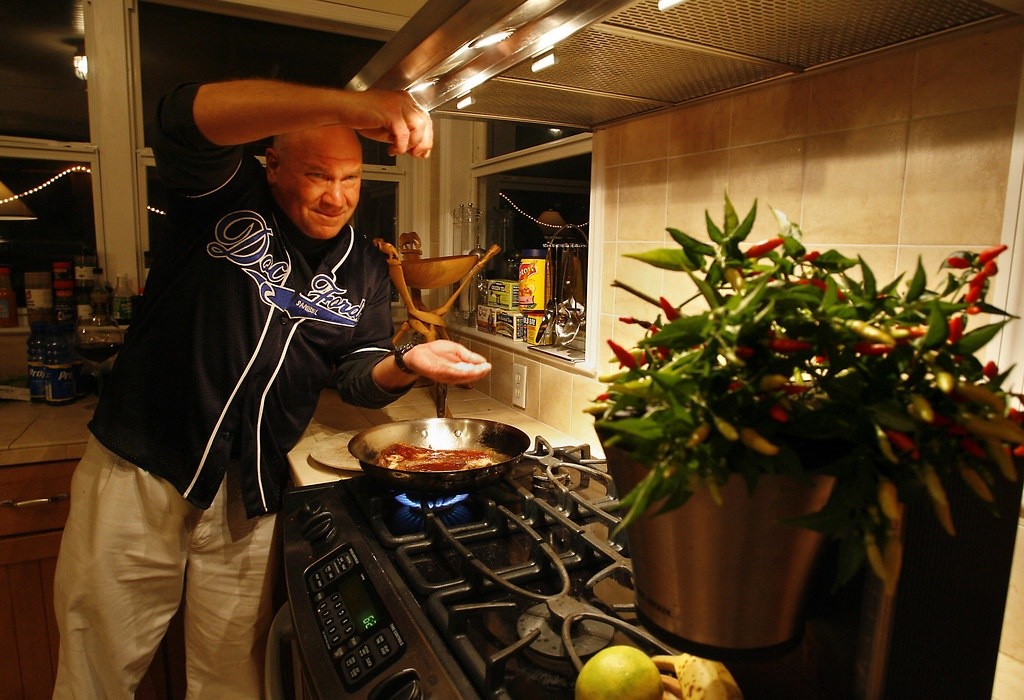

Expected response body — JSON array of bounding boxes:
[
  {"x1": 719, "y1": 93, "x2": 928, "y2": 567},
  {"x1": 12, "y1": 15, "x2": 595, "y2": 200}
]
[{"x1": 583, "y1": 235, "x2": 1023, "y2": 586}]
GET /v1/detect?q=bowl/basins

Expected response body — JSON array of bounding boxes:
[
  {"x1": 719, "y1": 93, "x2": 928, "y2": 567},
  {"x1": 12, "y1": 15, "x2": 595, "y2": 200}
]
[{"x1": 400, "y1": 255, "x2": 476, "y2": 288}]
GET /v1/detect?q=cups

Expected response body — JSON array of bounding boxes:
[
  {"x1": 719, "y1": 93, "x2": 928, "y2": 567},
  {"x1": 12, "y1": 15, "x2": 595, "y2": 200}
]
[
  {"x1": 24, "y1": 272, "x2": 51, "y2": 325},
  {"x1": 52, "y1": 272, "x2": 75, "y2": 322}
]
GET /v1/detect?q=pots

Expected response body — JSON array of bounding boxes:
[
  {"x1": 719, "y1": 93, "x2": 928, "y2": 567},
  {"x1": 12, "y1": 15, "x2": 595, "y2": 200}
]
[{"x1": 348, "y1": 419, "x2": 532, "y2": 496}]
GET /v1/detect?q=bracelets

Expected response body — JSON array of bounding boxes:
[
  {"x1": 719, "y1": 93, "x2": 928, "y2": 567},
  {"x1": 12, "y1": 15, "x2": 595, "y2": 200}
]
[{"x1": 394, "y1": 342, "x2": 415, "y2": 374}]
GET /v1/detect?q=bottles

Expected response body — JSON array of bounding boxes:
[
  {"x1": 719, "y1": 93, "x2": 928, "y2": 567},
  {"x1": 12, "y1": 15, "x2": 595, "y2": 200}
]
[
  {"x1": 518, "y1": 249, "x2": 554, "y2": 316},
  {"x1": 28, "y1": 321, "x2": 48, "y2": 402},
  {"x1": 90, "y1": 268, "x2": 109, "y2": 319},
  {"x1": 0, "y1": 268, "x2": 18, "y2": 328},
  {"x1": 45, "y1": 322, "x2": 76, "y2": 404},
  {"x1": 75, "y1": 278, "x2": 94, "y2": 320},
  {"x1": 111, "y1": 273, "x2": 135, "y2": 326}
]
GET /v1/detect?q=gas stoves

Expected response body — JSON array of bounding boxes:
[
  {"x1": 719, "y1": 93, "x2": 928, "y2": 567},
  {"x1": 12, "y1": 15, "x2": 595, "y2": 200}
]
[{"x1": 265, "y1": 443, "x2": 828, "y2": 700}]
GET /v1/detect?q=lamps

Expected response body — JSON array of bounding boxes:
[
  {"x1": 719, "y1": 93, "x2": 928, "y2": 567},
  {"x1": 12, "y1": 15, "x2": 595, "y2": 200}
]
[
  {"x1": 405, "y1": 77, "x2": 439, "y2": 92},
  {"x1": 530, "y1": 46, "x2": 560, "y2": 74},
  {"x1": 468, "y1": 27, "x2": 516, "y2": 49},
  {"x1": 0, "y1": 180, "x2": 38, "y2": 220},
  {"x1": 658, "y1": 0, "x2": 686, "y2": 12},
  {"x1": 456, "y1": 90, "x2": 476, "y2": 110},
  {"x1": 72, "y1": 40, "x2": 88, "y2": 80}
]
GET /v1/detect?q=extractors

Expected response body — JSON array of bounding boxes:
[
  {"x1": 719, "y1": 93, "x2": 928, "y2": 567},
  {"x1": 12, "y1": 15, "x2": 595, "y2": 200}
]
[{"x1": 345, "y1": 1, "x2": 1024, "y2": 133}]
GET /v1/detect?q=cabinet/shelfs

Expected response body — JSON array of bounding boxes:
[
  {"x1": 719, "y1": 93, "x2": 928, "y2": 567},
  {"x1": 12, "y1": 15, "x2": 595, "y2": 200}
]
[{"x1": 0, "y1": 458, "x2": 189, "y2": 700}]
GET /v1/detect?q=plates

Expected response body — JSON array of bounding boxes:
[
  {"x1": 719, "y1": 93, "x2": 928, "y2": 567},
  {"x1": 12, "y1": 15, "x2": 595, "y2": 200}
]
[{"x1": 310, "y1": 429, "x2": 366, "y2": 470}]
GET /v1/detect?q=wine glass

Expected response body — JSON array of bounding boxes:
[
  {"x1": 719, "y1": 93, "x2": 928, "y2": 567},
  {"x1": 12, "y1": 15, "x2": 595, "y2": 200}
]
[{"x1": 76, "y1": 315, "x2": 121, "y2": 411}]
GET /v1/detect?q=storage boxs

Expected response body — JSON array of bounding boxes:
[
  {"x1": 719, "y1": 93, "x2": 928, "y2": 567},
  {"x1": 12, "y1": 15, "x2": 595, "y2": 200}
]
[{"x1": 477, "y1": 280, "x2": 524, "y2": 342}]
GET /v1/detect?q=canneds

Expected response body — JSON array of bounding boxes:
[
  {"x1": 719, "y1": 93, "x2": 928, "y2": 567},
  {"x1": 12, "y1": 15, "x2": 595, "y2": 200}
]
[
  {"x1": 476, "y1": 248, "x2": 556, "y2": 346},
  {"x1": 23, "y1": 254, "x2": 97, "y2": 322}
]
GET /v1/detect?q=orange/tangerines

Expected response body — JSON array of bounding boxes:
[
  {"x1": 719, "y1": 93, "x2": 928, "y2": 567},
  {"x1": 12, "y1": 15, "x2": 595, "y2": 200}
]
[{"x1": 576, "y1": 645, "x2": 664, "y2": 700}]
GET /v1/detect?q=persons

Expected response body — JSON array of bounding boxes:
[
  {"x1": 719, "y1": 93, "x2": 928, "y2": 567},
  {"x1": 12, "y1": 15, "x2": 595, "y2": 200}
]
[{"x1": 52, "y1": 78, "x2": 492, "y2": 700}]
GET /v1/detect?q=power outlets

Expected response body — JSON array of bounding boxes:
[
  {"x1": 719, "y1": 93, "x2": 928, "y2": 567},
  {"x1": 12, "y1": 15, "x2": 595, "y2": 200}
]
[{"x1": 512, "y1": 363, "x2": 527, "y2": 408}]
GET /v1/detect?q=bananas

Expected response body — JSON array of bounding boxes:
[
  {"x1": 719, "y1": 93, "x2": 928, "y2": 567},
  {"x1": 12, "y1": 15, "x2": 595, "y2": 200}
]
[{"x1": 651, "y1": 652, "x2": 744, "y2": 700}]
